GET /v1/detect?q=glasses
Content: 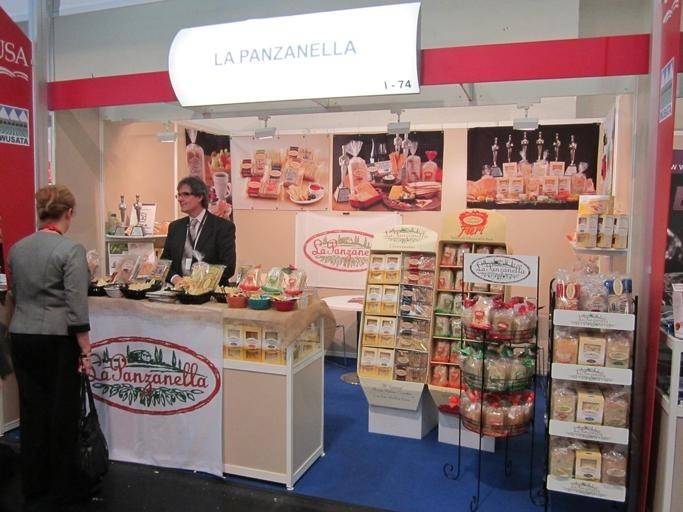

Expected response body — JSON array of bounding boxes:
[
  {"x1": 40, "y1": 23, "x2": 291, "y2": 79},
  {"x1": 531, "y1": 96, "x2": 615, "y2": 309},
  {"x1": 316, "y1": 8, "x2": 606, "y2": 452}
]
[{"x1": 176, "y1": 192, "x2": 192, "y2": 198}]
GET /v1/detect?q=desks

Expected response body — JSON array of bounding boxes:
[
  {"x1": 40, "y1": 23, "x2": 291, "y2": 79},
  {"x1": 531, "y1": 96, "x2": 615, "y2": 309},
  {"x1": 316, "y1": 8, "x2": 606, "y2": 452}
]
[{"x1": 320, "y1": 292, "x2": 365, "y2": 387}]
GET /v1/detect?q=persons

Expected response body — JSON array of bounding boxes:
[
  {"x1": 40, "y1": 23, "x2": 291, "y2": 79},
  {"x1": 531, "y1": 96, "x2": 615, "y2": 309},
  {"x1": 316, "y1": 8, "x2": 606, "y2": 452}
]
[
  {"x1": 6, "y1": 182, "x2": 91, "y2": 512},
  {"x1": 157, "y1": 176, "x2": 237, "y2": 288}
]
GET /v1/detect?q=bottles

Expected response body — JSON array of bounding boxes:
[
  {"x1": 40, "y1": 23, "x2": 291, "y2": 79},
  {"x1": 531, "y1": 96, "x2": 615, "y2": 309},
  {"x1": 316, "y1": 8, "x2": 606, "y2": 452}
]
[{"x1": 108, "y1": 214, "x2": 116, "y2": 235}]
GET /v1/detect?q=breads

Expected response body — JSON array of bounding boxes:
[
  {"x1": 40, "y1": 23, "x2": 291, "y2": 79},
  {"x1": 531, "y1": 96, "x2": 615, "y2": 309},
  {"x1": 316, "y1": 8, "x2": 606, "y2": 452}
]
[
  {"x1": 553, "y1": 332, "x2": 630, "y2": 368},
  {"x1": 550, "y1": 440, "x2": 625, "y2": 487},
  {"x1": 406, "y1": 157, "x2": 438, "y2": 182},
  {"x1": 555, "y1": 280, "x2": 634, "y2": 314},
  {"x1": 349, "y1": 162, "x2": 369, "y2": 190},
  {"x1": 552, "y1": 384, "x2": 627, "y2": 429}
]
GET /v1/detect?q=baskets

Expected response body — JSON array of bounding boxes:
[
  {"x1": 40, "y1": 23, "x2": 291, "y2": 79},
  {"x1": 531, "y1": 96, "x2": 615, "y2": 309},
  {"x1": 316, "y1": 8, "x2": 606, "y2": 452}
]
[
  {"x1": 104, "y1": 288, "x2": 123, "y2": 298},
  {"x1": 211, "y1": 293, "x2": 227, "y2": 303},
  {"x1": 248, "y1": 300, "x2": 270, "y2": 310},
  {"x1": 273, "y1": 300, "x2": 296, "y2": 312},
  {"x1": 88, "y1": 284, "x2": 112, "y2": 294},
  {"x1": 176, "y1": 291, "x2": 211, "y2": 304},
  {"x1": 225, "y1": 297, "x2": 247, "y2": 308},
  {"x1": 120, "y1": 284, "x2": 161, "y2": 300}
]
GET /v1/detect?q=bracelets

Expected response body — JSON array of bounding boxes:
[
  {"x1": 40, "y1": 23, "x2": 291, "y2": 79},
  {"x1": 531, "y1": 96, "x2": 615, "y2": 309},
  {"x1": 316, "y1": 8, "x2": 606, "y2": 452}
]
[{"x1": 79, "y1": 352, "x2": 93, "y2": 358}]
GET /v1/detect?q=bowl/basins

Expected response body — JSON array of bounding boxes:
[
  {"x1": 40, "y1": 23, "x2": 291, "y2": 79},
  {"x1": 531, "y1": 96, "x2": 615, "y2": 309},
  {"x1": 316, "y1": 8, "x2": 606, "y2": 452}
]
[
  {"x1": 226, "y1": 293, "x2": 298, "y2": 312},
  {"x1": 85, "y1": 278, "x2": 240, "y2": 306},
  {"x1": 348, "y1": 186, "x2": 383, "y2": 209}
]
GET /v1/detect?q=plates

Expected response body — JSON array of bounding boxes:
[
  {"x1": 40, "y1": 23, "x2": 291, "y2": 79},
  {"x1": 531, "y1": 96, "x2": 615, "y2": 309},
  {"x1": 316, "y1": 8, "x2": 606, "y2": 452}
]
[
  {"x1": 382, "y1": 187, "x2": 441, "y2": 210},
  {"x1": 288, "y1": 185, "x2": 327, "y2": 205}
]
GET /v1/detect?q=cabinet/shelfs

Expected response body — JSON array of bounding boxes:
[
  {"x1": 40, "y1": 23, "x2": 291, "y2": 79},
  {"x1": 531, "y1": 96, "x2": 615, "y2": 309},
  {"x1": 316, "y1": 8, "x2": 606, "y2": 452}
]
[{"x1": 354, "y1": 207, "x2": 510, "y2": 456}]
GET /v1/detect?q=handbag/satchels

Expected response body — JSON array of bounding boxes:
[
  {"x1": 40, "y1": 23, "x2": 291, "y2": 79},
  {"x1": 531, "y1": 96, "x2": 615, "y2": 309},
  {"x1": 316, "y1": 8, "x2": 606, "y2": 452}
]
[{"x1": 71, "y1": 411, "x2": 109, "y2": 484}]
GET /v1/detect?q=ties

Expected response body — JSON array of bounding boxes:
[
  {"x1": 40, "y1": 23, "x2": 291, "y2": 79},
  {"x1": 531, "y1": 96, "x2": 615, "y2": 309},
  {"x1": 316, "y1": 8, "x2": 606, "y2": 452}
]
[{"x1": 181, "y1": 219, "x2": 198, "y2": 275}]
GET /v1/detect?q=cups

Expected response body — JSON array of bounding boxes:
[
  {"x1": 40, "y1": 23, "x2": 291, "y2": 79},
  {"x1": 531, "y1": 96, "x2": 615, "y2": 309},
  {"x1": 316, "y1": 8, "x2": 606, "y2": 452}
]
[{"x1": 212, "y1": 171, "x2": 232, "y2": 201}]
